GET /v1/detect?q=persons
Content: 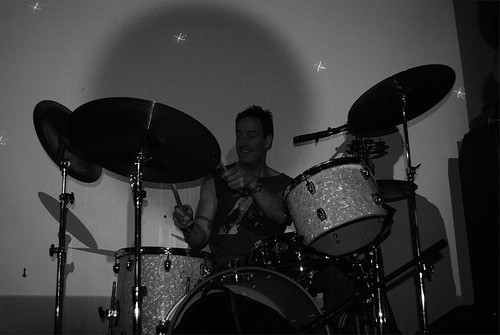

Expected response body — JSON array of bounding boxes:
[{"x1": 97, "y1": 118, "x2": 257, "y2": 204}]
[{"x1": 173, "y1": 106, "x2": 295, "y2": 268}]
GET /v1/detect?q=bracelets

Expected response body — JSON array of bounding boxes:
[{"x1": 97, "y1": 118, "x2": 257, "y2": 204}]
[{"x1": 249, "y1": 184, "x2": 264, "y2": 193}]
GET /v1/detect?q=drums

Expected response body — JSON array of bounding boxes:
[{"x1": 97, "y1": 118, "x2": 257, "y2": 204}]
[
  {"x1": 154, "y1": 266, "x2": 332, "y2": 335},
  {"x1": 109, "y1": 246, "x2": 221, "y2": 335},
  {"x1": 283, "y1": 156, "x2": 393, "y2": 263}
]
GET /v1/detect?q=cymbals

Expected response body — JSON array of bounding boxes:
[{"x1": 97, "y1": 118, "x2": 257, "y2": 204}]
[
  {"x1": 228, "y1": 231, "x2": 303, "y2": 268},
  {"x1": 73, "y1": 97, "x2": 222, "y2": 184},
  {"x1": 345, "y1": 64, "x2": 457, "y2": 135},
  {"x1": 32, "y1": 100, "x2": 102, "y2": 183},
  {"x1": 374, "y1": 176, "x2": 418, "y2": 202}
]
